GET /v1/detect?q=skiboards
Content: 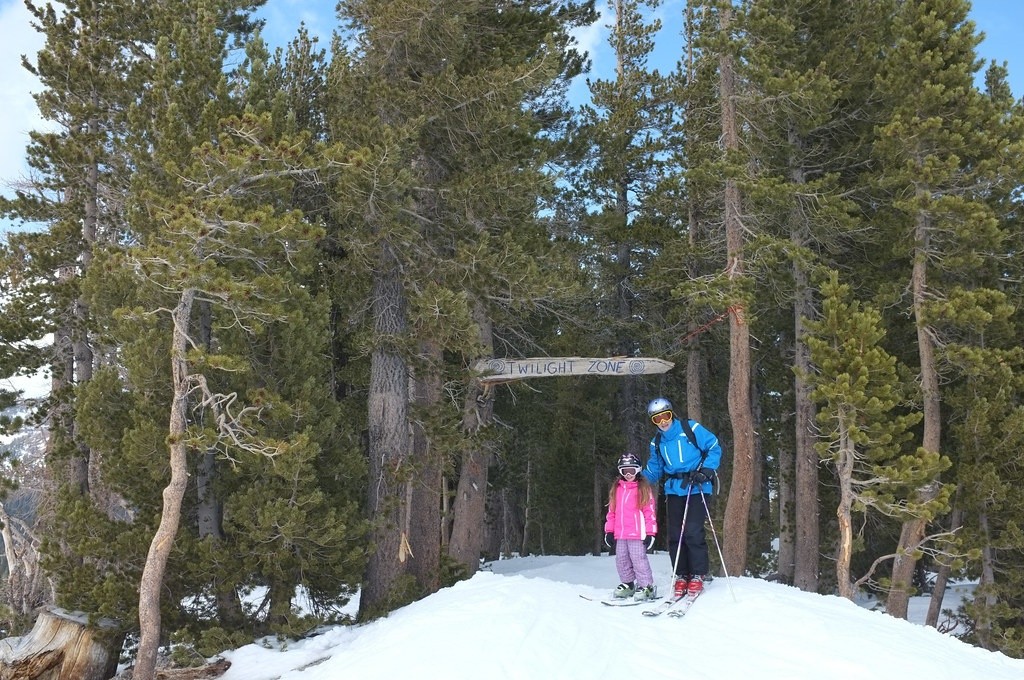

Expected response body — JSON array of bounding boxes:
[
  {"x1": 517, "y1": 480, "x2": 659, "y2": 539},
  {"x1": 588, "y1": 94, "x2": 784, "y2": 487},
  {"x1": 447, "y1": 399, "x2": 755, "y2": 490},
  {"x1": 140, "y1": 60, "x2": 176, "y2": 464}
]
[{"x1": 577, "y1": 576, "x2": 714, "y2": 620}]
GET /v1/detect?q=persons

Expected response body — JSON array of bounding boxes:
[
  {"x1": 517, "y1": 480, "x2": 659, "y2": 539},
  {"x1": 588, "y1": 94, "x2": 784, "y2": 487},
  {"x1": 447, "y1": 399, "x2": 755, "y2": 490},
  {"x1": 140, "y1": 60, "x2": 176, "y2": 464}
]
[
  {"x1": 604, "y1": 452, "x2": 658, "y2": 601},
  {"x1": 640, "y1": 398, "x2": 722, "y2": 598}
]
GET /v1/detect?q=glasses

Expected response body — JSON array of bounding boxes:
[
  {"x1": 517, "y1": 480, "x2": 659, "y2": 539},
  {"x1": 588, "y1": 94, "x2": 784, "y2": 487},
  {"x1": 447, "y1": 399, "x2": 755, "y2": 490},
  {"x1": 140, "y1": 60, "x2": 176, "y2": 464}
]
[
  {"x1": 652, "y1": 410, "x2": 672, "y2": 426},
  {"x1": 619, "y1": 466, "x2": 638, "y2": 477}
]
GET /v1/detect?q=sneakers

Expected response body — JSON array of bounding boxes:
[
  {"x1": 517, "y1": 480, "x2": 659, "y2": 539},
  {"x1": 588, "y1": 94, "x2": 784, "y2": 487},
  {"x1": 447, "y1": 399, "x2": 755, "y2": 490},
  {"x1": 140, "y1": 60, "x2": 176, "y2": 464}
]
[
  {"x1": 687, "y1": 574, "x2": 703, "y2": 595},
  {"x1": 633, "y1": 586, "x2": 654, "y2": 600},
  {"x1": 614, "y1": 582, "x2": 635, "y2": 597},
  {"x1": 675, "y1": 575, "x2": 688, "y2": 596}
]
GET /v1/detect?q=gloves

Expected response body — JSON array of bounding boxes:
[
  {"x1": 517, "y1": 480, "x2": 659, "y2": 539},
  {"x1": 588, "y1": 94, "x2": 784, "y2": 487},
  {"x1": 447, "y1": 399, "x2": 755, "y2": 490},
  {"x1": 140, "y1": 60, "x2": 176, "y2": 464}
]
[
  {"x1": 643, "y1": 535, "x2": 656, "y2": 551},
  {"x1": 693, "y1": 468, "x2": 714, "y2": 485},
  {"x1": 604, "y1": 533, "x2": 615, "y2": 549}
]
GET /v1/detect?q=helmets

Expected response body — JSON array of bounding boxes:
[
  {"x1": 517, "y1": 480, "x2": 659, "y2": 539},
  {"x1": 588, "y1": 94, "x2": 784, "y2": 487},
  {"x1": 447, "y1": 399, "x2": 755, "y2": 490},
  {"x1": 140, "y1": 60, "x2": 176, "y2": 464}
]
[
  {"x1": 618, "y1": 452, "x2": 641, "y2": 469},
  {"x1": 648, "y1": 398, "x2": 673, "y2": 417}
]
